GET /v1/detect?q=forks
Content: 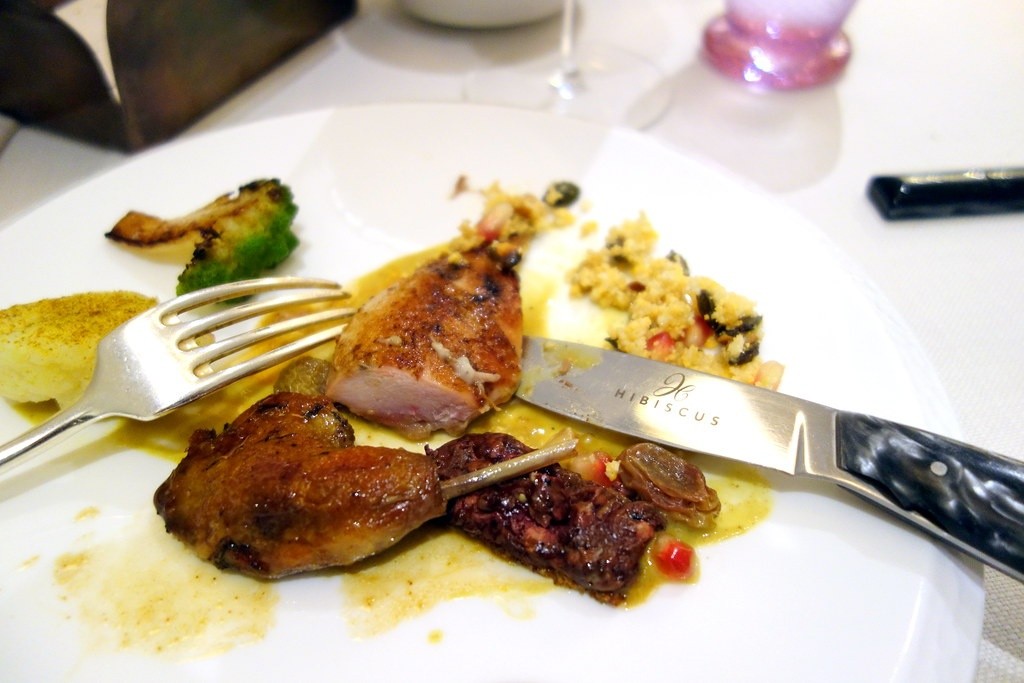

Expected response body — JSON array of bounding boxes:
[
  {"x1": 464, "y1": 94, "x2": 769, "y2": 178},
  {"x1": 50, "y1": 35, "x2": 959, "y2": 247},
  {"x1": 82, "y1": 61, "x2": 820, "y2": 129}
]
[{"x1": 0, "y1": 275, "x2": 360, "y2": 470}]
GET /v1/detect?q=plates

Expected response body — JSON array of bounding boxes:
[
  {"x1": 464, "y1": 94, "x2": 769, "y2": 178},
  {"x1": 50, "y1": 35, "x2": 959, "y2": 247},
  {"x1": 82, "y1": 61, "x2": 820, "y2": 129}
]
[{"x1": 0, "y1": 104, "x2": 987, "y2": 683}]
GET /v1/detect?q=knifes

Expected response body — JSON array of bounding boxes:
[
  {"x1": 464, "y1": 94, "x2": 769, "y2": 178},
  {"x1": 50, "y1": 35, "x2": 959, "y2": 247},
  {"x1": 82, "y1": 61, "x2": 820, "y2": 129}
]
[{"x1": 517, "y1": 336, "x2": 1023, "y2": 583}]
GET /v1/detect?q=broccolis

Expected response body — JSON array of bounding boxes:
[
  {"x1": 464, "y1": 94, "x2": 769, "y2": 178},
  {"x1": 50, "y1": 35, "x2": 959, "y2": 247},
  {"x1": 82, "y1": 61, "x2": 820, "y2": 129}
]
[{"x1": 105, "y1": 178, "x2": 298, "y2": 306}]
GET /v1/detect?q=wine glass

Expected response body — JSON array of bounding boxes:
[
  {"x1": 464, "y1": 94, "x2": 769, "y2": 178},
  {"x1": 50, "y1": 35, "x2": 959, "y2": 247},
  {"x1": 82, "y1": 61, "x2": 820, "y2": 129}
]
[{"x1": 516, "y1": 0, "x2": 666, "y2": 131}]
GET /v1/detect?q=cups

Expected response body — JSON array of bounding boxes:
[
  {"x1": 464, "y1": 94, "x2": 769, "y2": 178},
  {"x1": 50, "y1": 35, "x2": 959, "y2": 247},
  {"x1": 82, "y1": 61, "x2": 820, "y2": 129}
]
[
  {"x1": 700, "y1": 0, "x2": 858, "y2": 88},
  {"x1": 402, "y1": 0, "x2": 565, "y2": 27}
]
